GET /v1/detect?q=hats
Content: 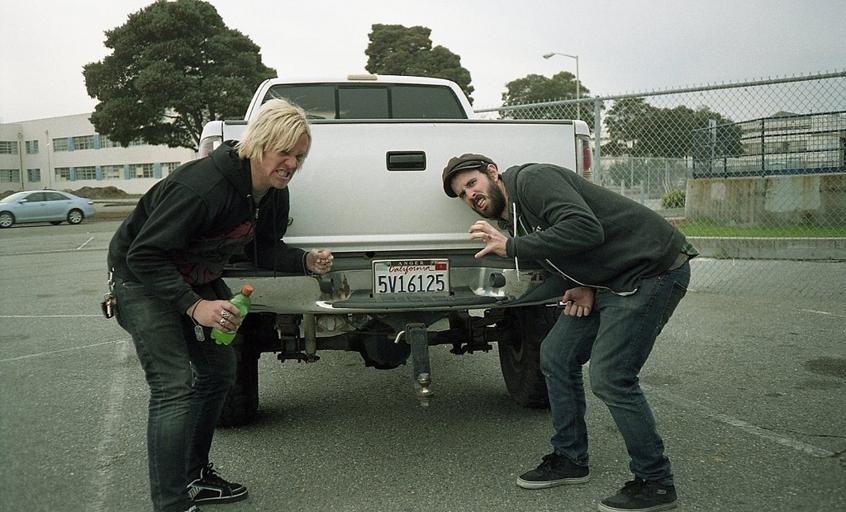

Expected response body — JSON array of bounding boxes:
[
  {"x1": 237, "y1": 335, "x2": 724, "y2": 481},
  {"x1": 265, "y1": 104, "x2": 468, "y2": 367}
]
[{"x1": 441, "y1": 152, "x2": 498, "y2": 199}]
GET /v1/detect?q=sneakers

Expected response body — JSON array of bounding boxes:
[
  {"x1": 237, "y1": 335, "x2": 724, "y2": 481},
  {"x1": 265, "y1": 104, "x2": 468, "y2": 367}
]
[
  {"x1": 515, "y1": 451, "x2": 593, "y2": 491},
  {"x1": 184, "y1": 461, "x2": 249, "y2": 512},
  {"x1": 597, "y1": 473, "x2": 680, "y2": 512}
]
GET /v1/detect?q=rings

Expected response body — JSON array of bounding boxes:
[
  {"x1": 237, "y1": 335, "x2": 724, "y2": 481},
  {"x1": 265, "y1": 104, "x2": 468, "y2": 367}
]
[
  {"x1": 486, "y1": 233, "x2": 494, "y2": 241},
  {"x1": 219, "y1": 318, "x2": 225, "y2": 325},
  {"x1": 324, "y1": 257, "x2": 330, "y2": 264},
  {"x1": 221, "y1": 308, "x2": 232, "y2": 319}
]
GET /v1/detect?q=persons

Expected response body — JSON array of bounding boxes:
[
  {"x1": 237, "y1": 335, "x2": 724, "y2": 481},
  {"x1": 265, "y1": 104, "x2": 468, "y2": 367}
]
[
  {"x1": 98, "y1": 97, "x2": 334, "y2": 510},
  {"x1": 441, "y1": 152, "x2": 700, "y2": 511}
]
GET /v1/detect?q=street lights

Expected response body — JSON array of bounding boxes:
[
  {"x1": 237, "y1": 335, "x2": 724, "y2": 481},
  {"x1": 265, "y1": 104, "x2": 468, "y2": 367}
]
[{"x1": 542, "y1": 51, "x2": 581, "y2": 121}]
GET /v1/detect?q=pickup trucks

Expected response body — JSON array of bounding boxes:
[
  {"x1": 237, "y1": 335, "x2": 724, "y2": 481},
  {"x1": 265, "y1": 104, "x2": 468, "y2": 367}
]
[{"x1": 193, "y1": 73, "x2": 596, "y2": 429}]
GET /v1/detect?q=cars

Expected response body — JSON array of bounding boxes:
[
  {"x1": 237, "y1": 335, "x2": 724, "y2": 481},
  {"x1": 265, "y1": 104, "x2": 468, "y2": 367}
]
[{"x1": 0, "y1": 188, "x2": 97, "y2": 227}]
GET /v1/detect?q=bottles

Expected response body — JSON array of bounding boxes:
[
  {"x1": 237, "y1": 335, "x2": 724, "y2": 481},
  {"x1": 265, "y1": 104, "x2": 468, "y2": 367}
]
[{"x1": 210, "y1": 286, "x2": 253, "y2": 346}]
